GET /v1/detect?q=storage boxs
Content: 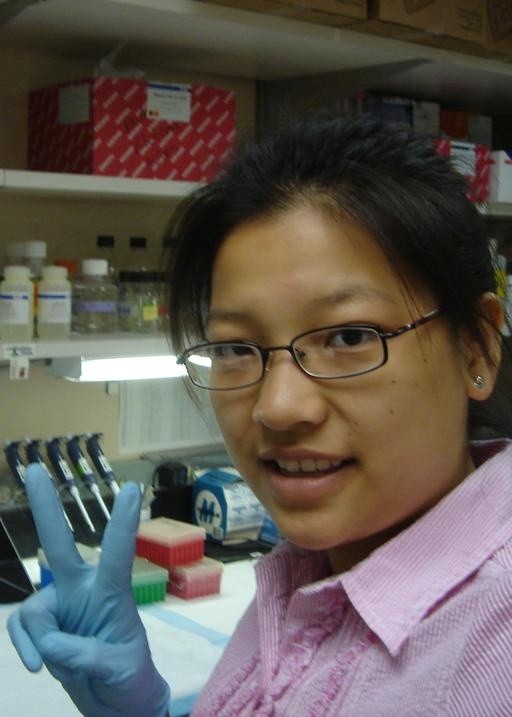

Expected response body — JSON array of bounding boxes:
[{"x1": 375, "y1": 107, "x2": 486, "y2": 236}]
[{"x1": 30, "y1": 75, "x2": 238, "y2": 186}]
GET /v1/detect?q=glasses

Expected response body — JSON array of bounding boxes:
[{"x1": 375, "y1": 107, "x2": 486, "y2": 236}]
[{"x1": 174, "y1": 304, "x2": 455, "y2": 393}]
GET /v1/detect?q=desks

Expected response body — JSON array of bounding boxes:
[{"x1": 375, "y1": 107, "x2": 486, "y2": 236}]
[{"x1": 1, "y1": 545, "x2": 266, "y2": 715}]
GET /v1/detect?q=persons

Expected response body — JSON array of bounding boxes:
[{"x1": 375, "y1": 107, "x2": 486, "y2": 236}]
[{"x1": 3, "y1": 108, "x2": 510, "y2": 717}]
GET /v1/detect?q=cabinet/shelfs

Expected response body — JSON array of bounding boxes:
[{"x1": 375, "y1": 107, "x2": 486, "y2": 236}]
[{"x1": 0, "y1": 0, "x2": 512, "y2": 363}]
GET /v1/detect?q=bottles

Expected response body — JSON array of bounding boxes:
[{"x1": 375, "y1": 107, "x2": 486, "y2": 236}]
[{"x1": 0, "y1": 232, "x2": 171, "y2": 342}]
